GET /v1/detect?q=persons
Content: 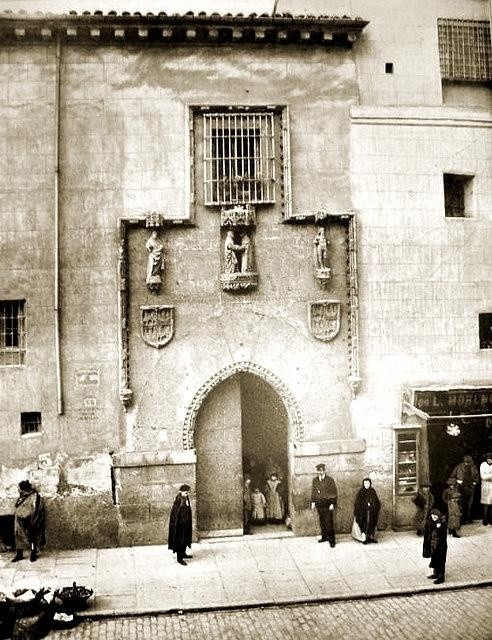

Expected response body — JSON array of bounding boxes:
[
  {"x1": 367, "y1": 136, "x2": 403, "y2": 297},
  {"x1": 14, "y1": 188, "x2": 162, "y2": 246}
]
[
  {"x1": 312, "y1": 226, "x2": 332, "y2": 275},
  {"x1": 238, "y1": 230, "x2": 254, "y2": 274},
  {"x1": 11, "y1": 479, "x2": 45, "y2": 563},
  {"x1": 144, "y1": 231, "x2": 166, "y2": 290},
  {"x1": 405, "y1": 452, "x2": 492, "y2": 585},
  {"x1": 167, "y1": 484, "x2": 193, "y2": 566},
  {"x1": 221, "y1": 229, "x2": 244, "y2": 276},
  {"x1": 309, "y1": 464, "x2": 339, "y2": 548},
  {"x1": 353, "y1": 477, "x2": 380, "y2": 544},
  {"x1": 241, "y1": 457, "x2": 287, "y2": 535}
]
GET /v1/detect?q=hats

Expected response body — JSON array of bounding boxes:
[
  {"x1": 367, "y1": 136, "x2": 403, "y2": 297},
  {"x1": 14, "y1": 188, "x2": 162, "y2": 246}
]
[
  {"x1": 315, "y1": 463, "x2": 326, "y2": 469},
  {"x1": 180, "y1": 484, "x2": 190, "y2": 491}
]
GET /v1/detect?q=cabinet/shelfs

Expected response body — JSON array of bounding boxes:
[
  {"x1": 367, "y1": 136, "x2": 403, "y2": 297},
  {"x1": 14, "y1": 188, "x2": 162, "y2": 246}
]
[{"x1": 393, "y1": 427, "x2": 420, "y2": 495}]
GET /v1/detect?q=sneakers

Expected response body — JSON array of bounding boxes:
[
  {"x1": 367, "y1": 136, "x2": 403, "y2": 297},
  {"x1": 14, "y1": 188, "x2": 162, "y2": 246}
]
[
  {"x1": 448, "y1": 517, "x2": 492, "y2": 538},
  {"x1": 368, "y1": 537, "x2": 378, "y2": 544},
  {"x1": 183, "y1": 554, "x2": 193, "y2": 559},
  {"x1": 318, "y1": 538, "x2": 328, "y2": 543},
  {"x1": 330, "y1": 539, "x2": 335, "y2": 548},
  {"x1": 434, "y1": 577, "x2": 445, "y2": 584},
  {"x1": 428, "y1": 574, "x2": 438, "y2": 580},
  {"x1": 363, "y1": 540, "x2": 368, "y2": 545},
  {"x1": 177, "y1": 559, "x2": 187, "y2": 566},
  {"x1": 11, "y1": 556, "x2": 23, "y2": 562},
  {"x1": 30, "y1": 552, "x2": 36, "y2": 562}
]
